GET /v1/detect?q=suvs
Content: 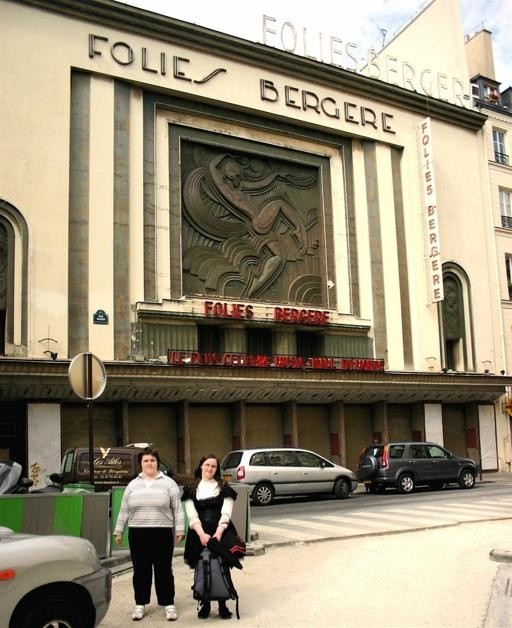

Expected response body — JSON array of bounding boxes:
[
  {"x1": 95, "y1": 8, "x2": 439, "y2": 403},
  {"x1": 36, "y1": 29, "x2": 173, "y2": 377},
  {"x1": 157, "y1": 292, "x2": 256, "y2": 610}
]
[{"x1": 355, "y1": 439, "x2": 482, "y2": 496}]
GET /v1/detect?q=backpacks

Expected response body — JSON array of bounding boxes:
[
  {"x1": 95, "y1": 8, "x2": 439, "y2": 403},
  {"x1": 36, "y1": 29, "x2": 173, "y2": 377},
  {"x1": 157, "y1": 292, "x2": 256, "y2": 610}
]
[{"x1": 191, "y1": 544, "x2": 237, "y2": 601}]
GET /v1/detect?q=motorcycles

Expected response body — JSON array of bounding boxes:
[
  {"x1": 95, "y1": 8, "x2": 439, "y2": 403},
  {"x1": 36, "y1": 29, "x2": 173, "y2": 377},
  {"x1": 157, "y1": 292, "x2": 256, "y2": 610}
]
[{"x1": 0, "y1": 455, "x2": 64, "y2": 497}]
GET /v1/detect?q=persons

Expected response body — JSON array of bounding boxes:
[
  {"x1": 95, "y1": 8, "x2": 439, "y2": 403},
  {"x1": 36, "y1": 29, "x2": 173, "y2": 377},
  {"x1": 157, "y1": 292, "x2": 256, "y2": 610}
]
[
  {"x1": 182, "y1": 452, "x2": 246, "y2": 616},
  {"x1": 114, "y1": 449, "x2": 185, "y2": 623},
  {"x1": 212, "y1": 153, "x2": 307, "y2": 297}
]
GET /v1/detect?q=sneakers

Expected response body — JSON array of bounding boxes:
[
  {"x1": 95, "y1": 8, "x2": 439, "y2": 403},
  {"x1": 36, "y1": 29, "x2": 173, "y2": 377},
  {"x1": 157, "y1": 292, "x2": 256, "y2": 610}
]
[
  {"x1": 165, "y1": 605, "x2": 178, "y2": 621},
  {"x1": 132, "y1": 604, "x2": 145, "y2": 620},
  {"x1": 198, "y1": 605, "x2": 211, "y2": 619},
  {"x1": 218, "y1": 605, "x2": 233, "y2": 619}
]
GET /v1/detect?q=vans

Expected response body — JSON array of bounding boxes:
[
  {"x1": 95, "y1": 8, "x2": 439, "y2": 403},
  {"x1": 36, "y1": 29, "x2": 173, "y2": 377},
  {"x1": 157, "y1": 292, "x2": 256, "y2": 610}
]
[
  {"x1": 218, "y1": 447, "x2": 361, "y2": 507},
  {"x1": 57, "y1": 444, "x2": 183, "y2": 501}
]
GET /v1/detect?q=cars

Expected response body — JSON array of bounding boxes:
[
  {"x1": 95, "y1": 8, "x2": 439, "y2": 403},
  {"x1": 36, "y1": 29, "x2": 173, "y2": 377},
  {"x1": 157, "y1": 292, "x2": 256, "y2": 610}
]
[{"x1": 0, "y1": 523, "x2": 114, "y2": 627}]
